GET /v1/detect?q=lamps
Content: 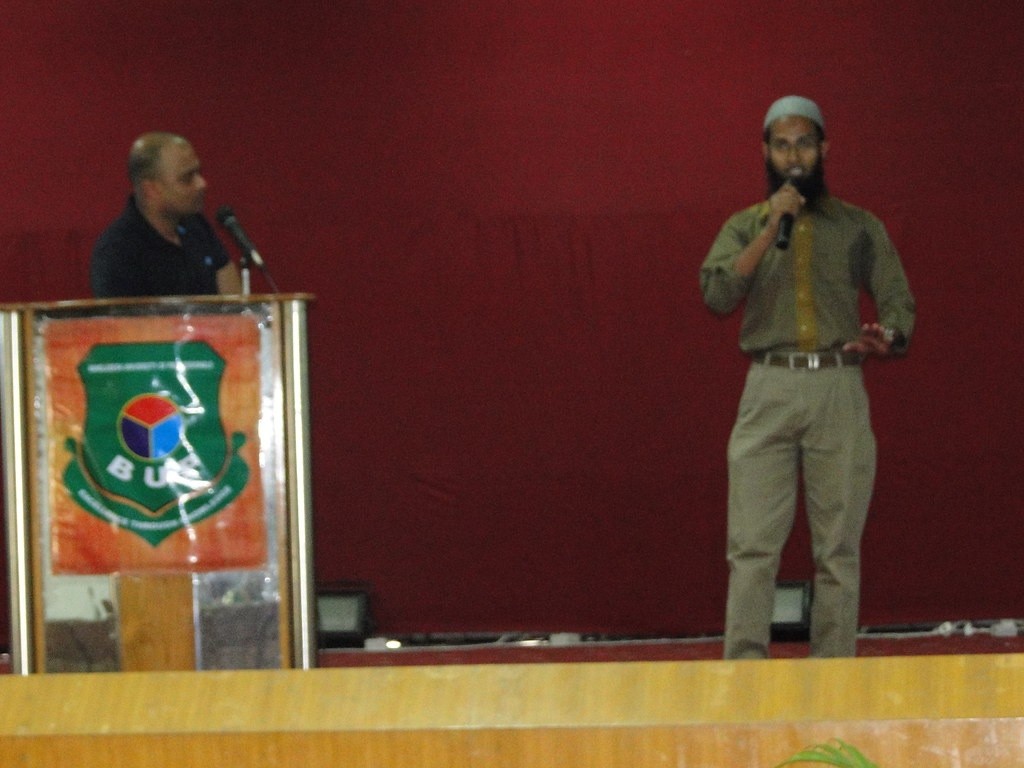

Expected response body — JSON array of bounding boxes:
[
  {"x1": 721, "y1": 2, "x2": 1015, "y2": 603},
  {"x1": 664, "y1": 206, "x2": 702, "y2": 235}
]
[
  {"x1": 771, "y1": 576, "x2": 815, "y2": 642},
  {"x1": 310, "y1": 582, "x2": 372, "y2": 652}
]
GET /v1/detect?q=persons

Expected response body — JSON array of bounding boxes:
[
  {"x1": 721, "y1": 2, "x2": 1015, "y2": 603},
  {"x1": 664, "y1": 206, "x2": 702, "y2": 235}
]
[
  {"x1": 701, "y1": 93, "x2": 915, "y2": 660},
  {"x1": 87, "y1": 132, "x2": 244, "y2": 298}
]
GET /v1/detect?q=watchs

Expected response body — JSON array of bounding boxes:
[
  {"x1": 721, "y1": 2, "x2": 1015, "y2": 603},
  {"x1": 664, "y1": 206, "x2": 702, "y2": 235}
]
[{"x1": 880, "y1": 326, "x2": 896, "y2": 337}]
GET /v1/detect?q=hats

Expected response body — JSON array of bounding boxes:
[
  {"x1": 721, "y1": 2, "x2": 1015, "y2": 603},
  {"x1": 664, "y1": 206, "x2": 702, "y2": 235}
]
[{"x1": 764, "y1": 95, "x2": 823, "y2": 128}]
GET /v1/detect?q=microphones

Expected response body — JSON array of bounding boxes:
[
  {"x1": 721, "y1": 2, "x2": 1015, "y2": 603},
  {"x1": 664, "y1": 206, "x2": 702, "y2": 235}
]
[
  {"x1": 775, "y1": 211, "x2": 793, "y2": 251},
  {"x1": 216, "y1": 207, "x2": 266, "y2": 271}
]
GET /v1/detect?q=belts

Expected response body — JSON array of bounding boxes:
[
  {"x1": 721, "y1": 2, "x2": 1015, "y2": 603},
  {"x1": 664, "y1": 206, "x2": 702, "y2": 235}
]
[{"x1": 753, "y1": 355, "x2": 860, "y2": 368}]
